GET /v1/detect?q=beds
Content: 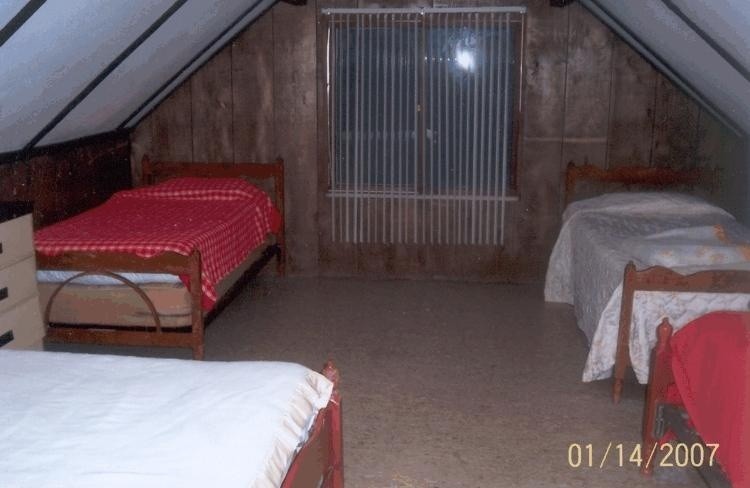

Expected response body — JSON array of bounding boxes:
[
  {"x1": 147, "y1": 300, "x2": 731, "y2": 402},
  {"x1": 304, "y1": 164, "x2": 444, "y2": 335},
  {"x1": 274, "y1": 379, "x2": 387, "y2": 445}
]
[
  {"x1": 563, "y1": 160, "x2": 750, "y2": 407},
  {"x1": 35, "y1": 150, "x2": 288, "y2": 361},
  {"x1": 639, "y1": 309, "x2": 750, "y2": 488},
  {"x1": 0, "y1": 348, "x2": 346, "y2": 488}
]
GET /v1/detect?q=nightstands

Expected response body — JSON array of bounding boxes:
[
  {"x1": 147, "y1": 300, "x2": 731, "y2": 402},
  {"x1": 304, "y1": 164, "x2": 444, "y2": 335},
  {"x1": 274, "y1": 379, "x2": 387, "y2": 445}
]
[{"x1": 0, "y1": 200, "x2": 49, "y2": 352}]
